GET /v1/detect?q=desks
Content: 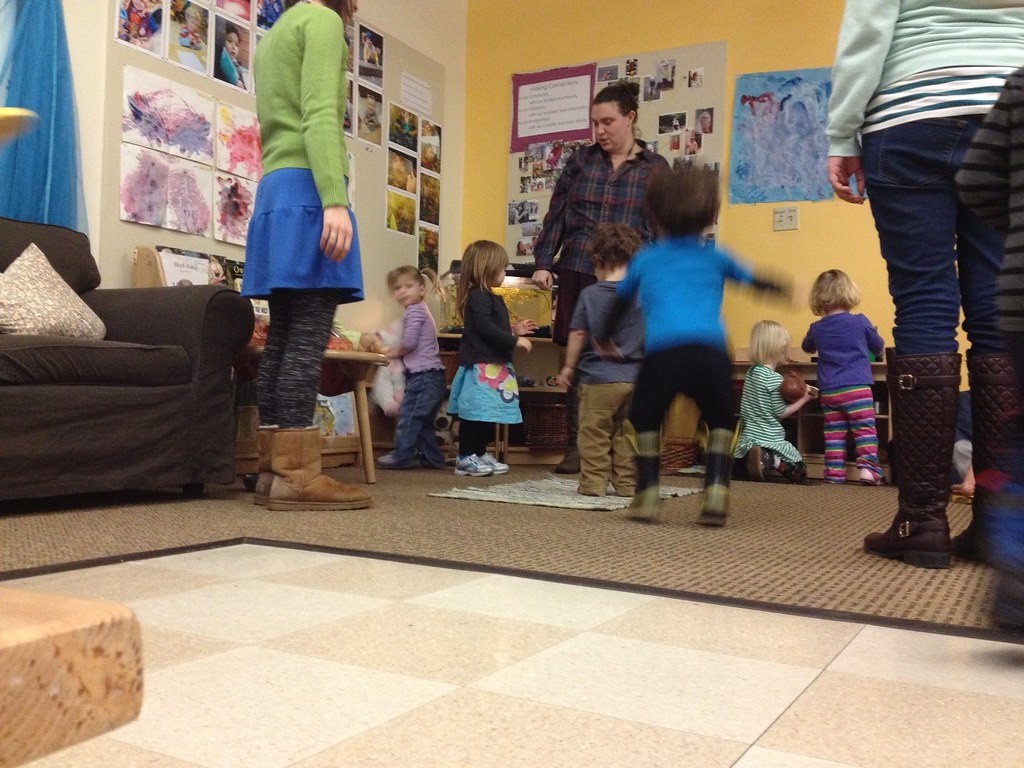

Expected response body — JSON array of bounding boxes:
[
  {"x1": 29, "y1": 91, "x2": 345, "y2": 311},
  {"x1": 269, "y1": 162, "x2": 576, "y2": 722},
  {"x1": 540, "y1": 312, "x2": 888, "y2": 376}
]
[{"x1": 247, "y1": 345, "x2": 390, "y2": 483}]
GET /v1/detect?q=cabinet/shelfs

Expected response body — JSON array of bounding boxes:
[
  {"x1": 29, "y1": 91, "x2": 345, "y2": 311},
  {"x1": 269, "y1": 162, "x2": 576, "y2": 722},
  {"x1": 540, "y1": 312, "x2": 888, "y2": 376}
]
[
  {"x1": 728, "y1": 359, "x2": 893, "y2": 485},
  {"x1": 434, "y1": 333, "x2": 573, "y2": 466}
]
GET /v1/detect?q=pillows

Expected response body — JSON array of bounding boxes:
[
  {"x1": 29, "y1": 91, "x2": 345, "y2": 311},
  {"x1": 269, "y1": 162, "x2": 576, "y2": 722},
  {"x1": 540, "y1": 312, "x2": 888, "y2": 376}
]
[{"x1": 0, "y1": 242, "x2": 108, "y2": 341}]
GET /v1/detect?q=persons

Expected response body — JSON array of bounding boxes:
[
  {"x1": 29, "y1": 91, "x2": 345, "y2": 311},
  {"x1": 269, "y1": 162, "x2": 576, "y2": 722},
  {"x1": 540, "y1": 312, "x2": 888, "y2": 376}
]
[
  {"x1": 826, "y1": 0, "x2": 1024, "y2": 632},
  {"x1": 240, "y1": 0, "x2": 373, "y2": 510},
  {"x1": 369, "y1": 266, "x2": 446, "y2": 468},
  {"x1": 446, "y1": 239, "x2": 538, "y2": 476},
  {"x1": 617, "y1": 161, "x2": 783, "y2": 525},
  {"x1": 802, "y1": 269, "x2": 886, "y2": 485},
  {"x1": 690, "y1": 71, "x2": 699, "y2": 84},
  {"x1": 120, "y1": 0, "x2": 150, "y2": 48},
  {"x1": 687, "y1": 137, "x2": 697, "y2": 154},
  {"x1": 179, "y1": 7, "x2": 203, "y2": 51},
  {"x1": 517, "y1": 142, "x2": 581, "y2": 256},
  {"x1": 700, "y1": 111, "x2": 711, "y2": 131},
  {"x1": 219, "y1": 22, "x2": 246, "y2": 89},
  {"x1": 364, "y1": 94, "x2": 380, "y2": 127},
  {"x1": 734, "y1": 320, "x2": 819, "y2": 481},
  {"x1": 661, "y1": 65, "x2": 669, "y2": 83},
  {"x1": 554, "y1": 222, "x2": 642, "y2": 497},
  {"x1": 364, "y1": 32, "x2": 379, "y2": 65},
  {"x1": 533, "y1": 87, "x2": 672, "y2": 473},
  {"x1": 672, "y1": 115, "x2": 680, "y2": 150}
]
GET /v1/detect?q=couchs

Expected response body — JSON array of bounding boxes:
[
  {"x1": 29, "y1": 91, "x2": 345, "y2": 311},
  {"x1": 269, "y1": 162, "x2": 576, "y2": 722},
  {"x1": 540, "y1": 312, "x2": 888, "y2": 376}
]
[{"x1": 0, "y1": 216, "x2": 254, "y2": 499}]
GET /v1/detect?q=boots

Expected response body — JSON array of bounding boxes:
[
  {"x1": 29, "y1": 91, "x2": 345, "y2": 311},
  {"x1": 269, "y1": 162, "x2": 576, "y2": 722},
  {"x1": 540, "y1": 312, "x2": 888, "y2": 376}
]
[
  {"x1": 622, "y1": 418, "x2": 663, "y2": 521},
  {"x1": 554, "y1": 385, "x2": 579, "y2": 473},
  {"x1": 953, "y1": 341, "x2": 1024, "y2": 578},
  {"x1": 748, "y1": 446, "x2": 774, "y2": 481},
  {"x1": 253, "y1": 425, "x2": 281, "y2": 505},
  {"x1": 267, "y1": 425, "x2": 371, "y2": 511},
  {"x1": 696, "y1": 419, "x2": 740, "y2": 525},
  {"x1": 864, "y1": 345, "x2": 966, "y2": 569}
]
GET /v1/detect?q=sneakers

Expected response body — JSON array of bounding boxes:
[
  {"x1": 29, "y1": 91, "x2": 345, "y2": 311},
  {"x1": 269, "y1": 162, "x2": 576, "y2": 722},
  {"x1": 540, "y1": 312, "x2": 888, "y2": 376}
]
[
  {"x1": 477, "y1": 454, "x2": 509, "y2": 474},
  {"x1": 454, "y1": 453, "x2": 493, "y2": 477}
]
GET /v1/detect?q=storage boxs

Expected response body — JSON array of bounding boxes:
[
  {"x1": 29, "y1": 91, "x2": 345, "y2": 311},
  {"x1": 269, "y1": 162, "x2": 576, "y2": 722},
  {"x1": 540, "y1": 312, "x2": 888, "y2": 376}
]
[{"x1": 319, "y1": 436, "x2": 361, "y2": 468}]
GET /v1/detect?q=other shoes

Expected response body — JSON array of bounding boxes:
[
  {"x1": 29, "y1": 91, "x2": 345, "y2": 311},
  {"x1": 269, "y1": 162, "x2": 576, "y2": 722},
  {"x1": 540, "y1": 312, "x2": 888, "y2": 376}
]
[
  {"x1": 860, "y1": 468, "x2": 881, "y2": 485},
  {"x1": 377, "y1": 451, "x2": 401, "y2": 468}
]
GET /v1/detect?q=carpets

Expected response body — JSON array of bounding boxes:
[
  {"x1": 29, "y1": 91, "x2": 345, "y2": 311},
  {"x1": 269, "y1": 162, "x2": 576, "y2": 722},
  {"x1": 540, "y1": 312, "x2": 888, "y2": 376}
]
[{"x1": 0, "y1": 457, "x2": 1024, "y2": 647}]
